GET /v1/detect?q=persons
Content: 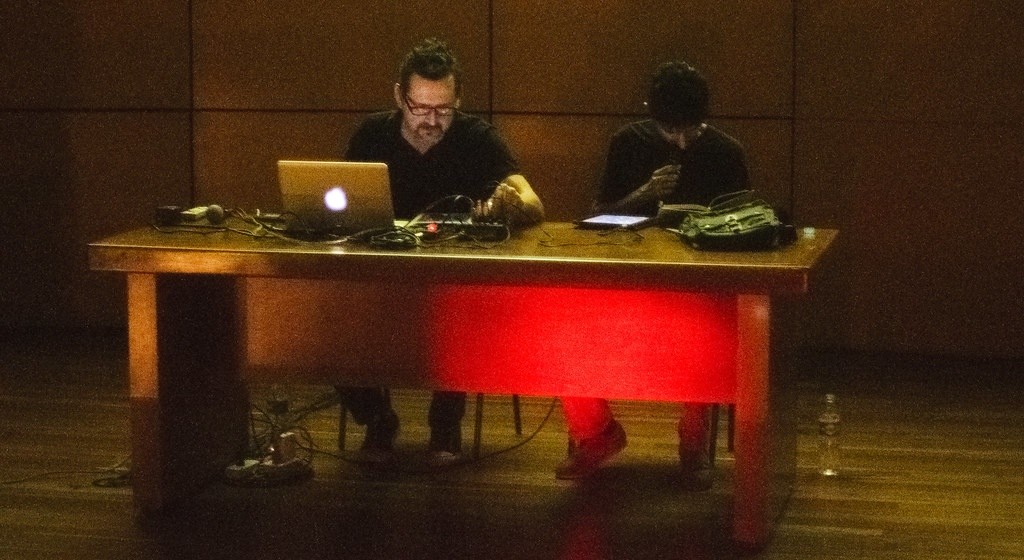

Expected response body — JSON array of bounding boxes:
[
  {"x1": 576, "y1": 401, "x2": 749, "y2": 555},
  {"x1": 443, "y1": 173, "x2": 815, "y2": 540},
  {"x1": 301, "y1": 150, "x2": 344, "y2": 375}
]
[
  {"x1": 343, "y1": 36, "x2": 546, "y2": 475},
  {"x1": 553, "y1": 61, "x2": 751, "y2": 487}
]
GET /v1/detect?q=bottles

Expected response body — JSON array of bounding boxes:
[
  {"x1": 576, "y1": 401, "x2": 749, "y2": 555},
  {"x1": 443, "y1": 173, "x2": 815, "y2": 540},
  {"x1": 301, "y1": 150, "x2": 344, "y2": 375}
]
[{"x1": 816, "y1": 394, "x2": 841, "y2": 476}]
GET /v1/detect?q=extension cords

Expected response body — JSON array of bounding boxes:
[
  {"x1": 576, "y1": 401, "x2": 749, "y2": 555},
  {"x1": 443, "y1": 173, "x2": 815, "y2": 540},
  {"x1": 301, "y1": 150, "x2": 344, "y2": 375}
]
[{"x1": 224, "y1": 458, "x2": 261, "y2": 482}]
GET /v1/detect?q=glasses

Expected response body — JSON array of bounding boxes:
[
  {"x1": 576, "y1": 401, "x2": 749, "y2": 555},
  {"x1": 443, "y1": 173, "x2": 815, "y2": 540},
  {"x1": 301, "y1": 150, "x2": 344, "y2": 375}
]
[
  {"x1": 401, "y1": 92, "x2": 459, "y2": 116},
  {"x1": 662, "y1": 123, "x2": 708, "y2": 137}
]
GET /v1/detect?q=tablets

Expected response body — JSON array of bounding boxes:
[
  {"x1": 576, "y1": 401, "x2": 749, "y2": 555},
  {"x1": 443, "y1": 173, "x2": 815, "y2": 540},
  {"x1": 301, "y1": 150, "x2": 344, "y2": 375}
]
[{"x1": 572, "y1": 211, "x2": 655, "y2": 230}]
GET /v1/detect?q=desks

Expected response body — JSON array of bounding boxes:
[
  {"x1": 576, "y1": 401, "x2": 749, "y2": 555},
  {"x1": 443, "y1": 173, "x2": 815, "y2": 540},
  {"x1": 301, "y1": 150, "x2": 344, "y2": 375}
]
[{"x1": 87, "y1": 214, "x2": 838, "y2": 548}]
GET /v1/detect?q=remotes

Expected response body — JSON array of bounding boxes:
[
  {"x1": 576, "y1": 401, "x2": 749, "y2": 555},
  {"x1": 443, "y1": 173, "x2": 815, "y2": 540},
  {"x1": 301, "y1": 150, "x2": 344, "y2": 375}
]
[{"x1": 182, "y1": 205, "x2": 209, "y2": 221}]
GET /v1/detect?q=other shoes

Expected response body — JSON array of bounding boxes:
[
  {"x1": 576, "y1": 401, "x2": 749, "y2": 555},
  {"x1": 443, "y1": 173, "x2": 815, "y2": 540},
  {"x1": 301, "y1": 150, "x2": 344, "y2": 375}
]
[
  {"x1": 554, "y1": 418, "x2": 628, "y2": 478},
  {"x1": 426, "y1": 425, "x2": 462, "y2": 467},
  {"x1": 361, "y1": 409, "x2": 401, "y2": 463},
  {"x1": 679, "y1": 442, "x2": 714, "y2": 488}
]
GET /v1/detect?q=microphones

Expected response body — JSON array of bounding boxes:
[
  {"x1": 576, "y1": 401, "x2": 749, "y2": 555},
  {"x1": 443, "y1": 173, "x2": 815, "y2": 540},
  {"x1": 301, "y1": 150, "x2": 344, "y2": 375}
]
[
  {"x1": 664, "y1": 140, "x2": 684, "y2": 166},
  {"x1": 206, "y1": 203, "x2": 235, "y2": 224}
]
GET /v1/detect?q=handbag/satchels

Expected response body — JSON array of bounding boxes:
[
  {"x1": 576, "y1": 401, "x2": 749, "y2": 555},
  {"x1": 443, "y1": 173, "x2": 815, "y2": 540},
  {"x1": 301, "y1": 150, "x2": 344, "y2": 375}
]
[{"x1": 680, "y1": 200, "x2": 783, "y2": 251}]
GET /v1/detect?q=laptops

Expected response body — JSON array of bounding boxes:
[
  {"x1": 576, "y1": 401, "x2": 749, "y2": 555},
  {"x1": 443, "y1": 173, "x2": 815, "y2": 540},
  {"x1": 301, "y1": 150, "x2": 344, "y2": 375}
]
[{"x1": 277, "y1": 159, "x2": 410, "y2": 237}]
